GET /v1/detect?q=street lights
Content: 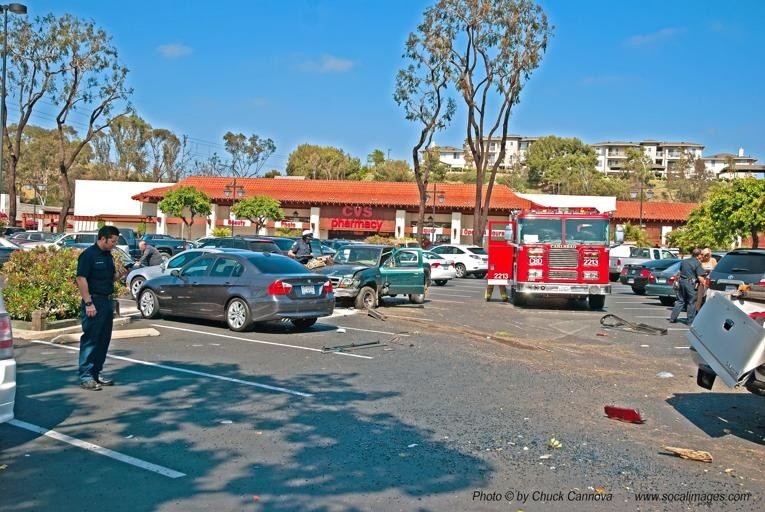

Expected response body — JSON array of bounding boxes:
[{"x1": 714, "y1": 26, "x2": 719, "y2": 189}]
[
  {"x1": 630, "y1": 181, "x2": 656, "y2": 231},
  {"x1": 425, "y1": 185, "x2": 445, "y2": 246},
  {"x1": 22, "y1": 176, "x2": 46, "y2": 229},
  {"x1": 223, "y1": 178, "x2": 245, "y2": 236}
]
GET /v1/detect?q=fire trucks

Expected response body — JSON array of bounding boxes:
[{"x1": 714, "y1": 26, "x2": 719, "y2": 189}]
[{"x1": 486, "y1": 206, "x2": 625, "y2": 309}]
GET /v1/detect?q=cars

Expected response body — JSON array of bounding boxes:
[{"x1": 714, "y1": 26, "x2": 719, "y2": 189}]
[
  {"x1": 136, "y1": 252, "x2": 335, "y2": 332},
  {"x1": 0, "y1": 225, "x2": 765, "y2": 310},
  {"x1": 0, "y1": 287, "x2": 15, "y2": 424},
  {"x1": 688, "y1": 291, "x2": 765, "y2": 396}
]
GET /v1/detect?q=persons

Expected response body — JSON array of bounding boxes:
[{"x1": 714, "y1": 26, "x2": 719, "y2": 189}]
[
  {"x1": 696, "y1": 247, "x2": 718, "y2": 313},
  {"x1": 133, "y1": 240, "x2": 164, "y2": 267},
  {"x1": 484, "y1": 285, "x2": 508, "y2": 302},
  {"x1": 136, "y1": 233, "x2": 142, "y2": 239},
  {"x1": 288, "y1": 229, "x2": 314, "y2": 264},
  {"x1": 75, "y1": 225, "x2": 129, "y2": 391},
  {"x1": 670, "y1": 248, "x2": 707, "y2": 325}
]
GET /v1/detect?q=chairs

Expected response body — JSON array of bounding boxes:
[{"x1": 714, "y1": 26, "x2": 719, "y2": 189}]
[{"x1": 222, "y1": 265, "x2": 234, "y2": 274}]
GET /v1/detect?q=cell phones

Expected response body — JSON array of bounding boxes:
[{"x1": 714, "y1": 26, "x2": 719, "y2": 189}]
[{"x1": 124, "y1": 263, "x2": 135, "y2": 270}]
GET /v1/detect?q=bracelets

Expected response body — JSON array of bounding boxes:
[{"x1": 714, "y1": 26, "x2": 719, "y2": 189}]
[{"x1": 85, "y1": 302, "x2": 93, "y2": 306}]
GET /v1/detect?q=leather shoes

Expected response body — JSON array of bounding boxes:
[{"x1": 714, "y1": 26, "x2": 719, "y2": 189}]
[
  {"x1": 94, "y1": 377, "x2": 112, "y2": 385},
  {"x1": 80, "y1": 381, "x2": 102, "y2": 390}
]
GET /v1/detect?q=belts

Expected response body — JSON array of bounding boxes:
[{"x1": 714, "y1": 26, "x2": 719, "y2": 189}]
[{"x1": 92, "y1": 294, "x2": 113, "y2": 300}]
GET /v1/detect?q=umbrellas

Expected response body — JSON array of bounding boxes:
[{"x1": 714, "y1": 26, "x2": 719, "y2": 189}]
[
  {"x1": 47, "y1": 220, "x2": 73, "y2": 229},
  {"x1": 16, "y1": 219, "x2": 38, "y2": 229}
]
